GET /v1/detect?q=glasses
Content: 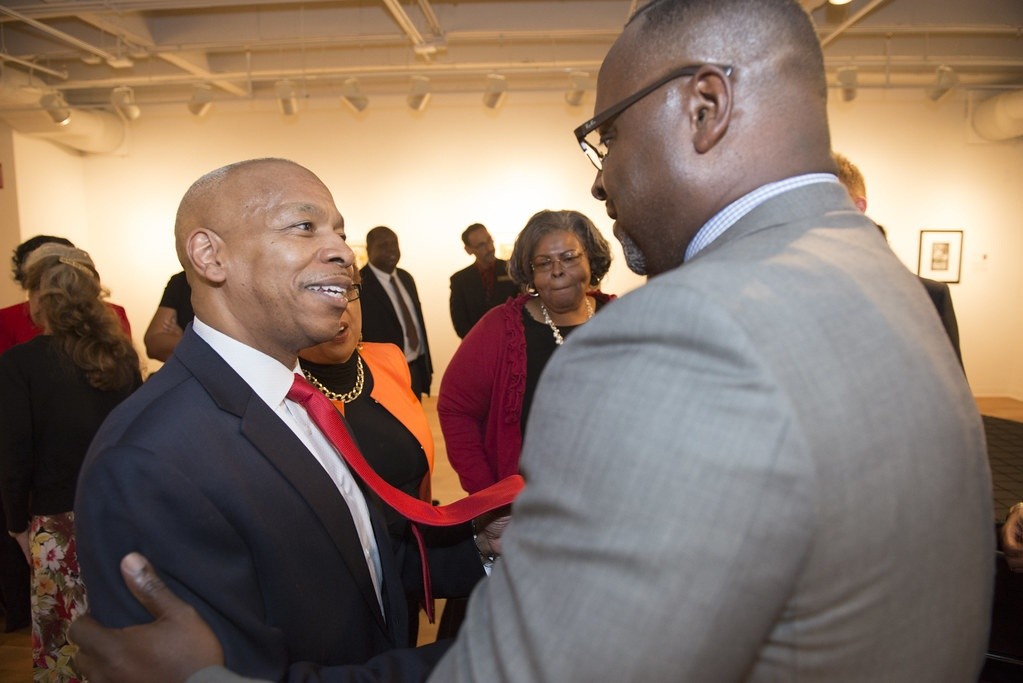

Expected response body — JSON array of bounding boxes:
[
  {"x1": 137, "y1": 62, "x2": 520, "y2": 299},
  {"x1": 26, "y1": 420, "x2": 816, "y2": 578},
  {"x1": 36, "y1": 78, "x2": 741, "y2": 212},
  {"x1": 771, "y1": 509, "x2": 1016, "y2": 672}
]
[
  {"x1": 573, "y1": 66, "x2": 733, "y2": 172},
  {"x1": 529, "y1": 247, "x2": 589, "y2": 273},
  {"x1": 346, "y1": 283, "x2": 362, "y2": 302}
]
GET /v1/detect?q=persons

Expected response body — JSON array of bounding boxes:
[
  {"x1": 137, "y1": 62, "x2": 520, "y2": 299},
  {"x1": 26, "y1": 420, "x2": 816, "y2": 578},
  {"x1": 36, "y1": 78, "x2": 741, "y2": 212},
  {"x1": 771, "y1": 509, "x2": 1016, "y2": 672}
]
[
  {"x1": 296, "y1": 261, "x2": 436, "y2": 505},
  {"x1": 1, "y1": 235, "x2": 144, "y2": 633},
  {"x1": 71, "y1": 0, "x2": 994, "y2": 682},
  {"x1": 144, "y1": 271, "x2": 194, "y2": 363},
  {"x1": 436, "y1": 209, "x2": 618, "y2": 497},
  {"x1": 449, "y1": 224, "x2": 521, "y2": 340},
  {"x1": 74, "y1": 158, "x2": 526, "y2": 680},
  {"x1": 830, "y1": 152, "x2": 969, "y2": 384},
  {"x1": 359, "y1": 227, "x2": 434, "y2": 405}
]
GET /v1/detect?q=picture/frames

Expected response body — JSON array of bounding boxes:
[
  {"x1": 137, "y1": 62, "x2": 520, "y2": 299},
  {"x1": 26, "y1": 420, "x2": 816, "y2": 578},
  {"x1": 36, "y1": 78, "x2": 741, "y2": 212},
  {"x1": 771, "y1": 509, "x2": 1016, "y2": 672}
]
[{"x1": 917, "y1": 230, "x2": 964, "y2": 284}]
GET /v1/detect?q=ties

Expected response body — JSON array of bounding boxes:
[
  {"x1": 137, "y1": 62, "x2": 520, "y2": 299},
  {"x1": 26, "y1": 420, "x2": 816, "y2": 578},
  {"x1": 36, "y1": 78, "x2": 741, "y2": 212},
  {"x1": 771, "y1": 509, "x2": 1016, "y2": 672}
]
[
  {"x1": 390, "y1": 276, "x2": 419, "y2": 351},
  {"x1": 483, "y1": 268, "x2": 492, "y2": 303},
  {"x1": 285, "y1": 374, "x2": 525, "y2": 625}
]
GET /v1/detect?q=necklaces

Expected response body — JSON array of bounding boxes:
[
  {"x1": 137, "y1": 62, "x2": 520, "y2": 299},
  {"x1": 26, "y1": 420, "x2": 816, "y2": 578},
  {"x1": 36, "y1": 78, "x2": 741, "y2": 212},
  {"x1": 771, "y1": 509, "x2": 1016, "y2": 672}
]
[
  {"x1": 538, "y1": 297, "x2": 595, "y2": 344},
  {"x1": 300, "y1": 355, "x2": 364, "y2": 404}
]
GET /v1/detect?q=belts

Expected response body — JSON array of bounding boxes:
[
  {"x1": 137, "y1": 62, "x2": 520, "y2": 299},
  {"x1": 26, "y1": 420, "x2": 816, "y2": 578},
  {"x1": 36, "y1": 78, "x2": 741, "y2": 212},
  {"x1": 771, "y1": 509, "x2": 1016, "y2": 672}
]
[{"x1": 408, "y1": 354, "x2": 424, "y2": 366}]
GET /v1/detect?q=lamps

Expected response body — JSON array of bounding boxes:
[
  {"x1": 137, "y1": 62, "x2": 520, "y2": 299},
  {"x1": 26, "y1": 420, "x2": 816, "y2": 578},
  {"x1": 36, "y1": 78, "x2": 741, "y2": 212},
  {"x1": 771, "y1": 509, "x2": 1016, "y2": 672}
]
[
  {"x1": 838, "y1": 67, "x2": 857, "y2": 103},
  {"x1": 927, "y1": 66, "x2": 956, "y2": 103},
  {"x1": 482, "y1": 72, "x2": 506, "y2": 109},
  {"x1": 275, "y1": 80, "x2": 297, "y2": 117},
  {"x1": 39, "y1": 90, "x2": 72, "y2": 127},
  {"x1": 112, "y1": 87, "x2": 140, "y2": 121},
  {"x1": 567, "y1": 71, "x2": 590, "y2": 106},
  {"x1": 186, "y1": 84, "x2": 215, "y2": 116},
  {"x1": 405, "y1": 76, "x2": 432, "y2": 112},
  {"x1": 338, "y1": 75, "x2": 370, "y2": 113}
]
